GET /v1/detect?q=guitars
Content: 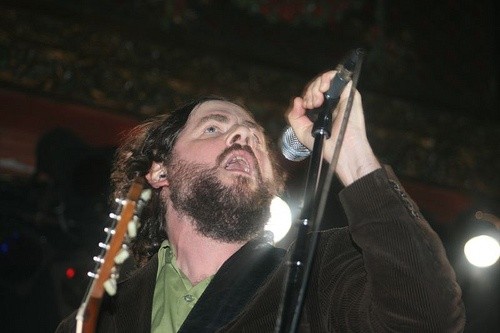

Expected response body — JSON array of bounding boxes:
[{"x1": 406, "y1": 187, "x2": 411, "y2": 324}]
[{"x1": 76, "y1": 177, "x2": 153, "y2": 333}]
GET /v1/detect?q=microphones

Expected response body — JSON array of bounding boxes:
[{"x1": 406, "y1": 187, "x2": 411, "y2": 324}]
[{"x1": 279, "y1": 50, "x2": 363, "y2": 161}]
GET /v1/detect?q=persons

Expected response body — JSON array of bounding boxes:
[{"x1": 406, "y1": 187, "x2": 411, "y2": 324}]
[{"x1": 57, "y1": 64, "x2": 468, "y2": 333}]
